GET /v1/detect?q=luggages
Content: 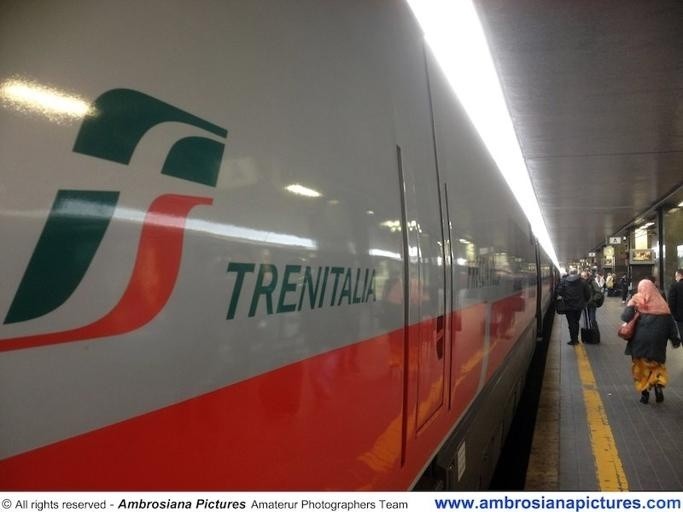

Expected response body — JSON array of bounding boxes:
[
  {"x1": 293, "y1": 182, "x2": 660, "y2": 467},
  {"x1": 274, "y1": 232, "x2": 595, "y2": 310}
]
[{"x1": 581, "y1": 327, "x2": 600, "y2": 345}]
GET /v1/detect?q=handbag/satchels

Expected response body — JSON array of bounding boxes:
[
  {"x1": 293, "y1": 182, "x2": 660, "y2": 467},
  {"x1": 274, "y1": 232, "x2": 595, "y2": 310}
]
[
  {"x1": 554, "y1": 298, "x2": 567, "y2": 315},
  {"x1": 618, "y1": 311, "x2": 640, "y2": 341},
  {"x1": 592, "y1": 293, "x2": 604, "y2": 308}
]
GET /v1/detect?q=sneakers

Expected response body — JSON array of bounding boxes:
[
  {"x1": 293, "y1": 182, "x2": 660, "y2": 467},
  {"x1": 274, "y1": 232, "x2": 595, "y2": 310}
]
[
  {"x1": 654, "y1": 384, "x2": 664, "y2": 403},
  {"x1": 567, "y1": 340, "x2": 579, "y2": 346},
  {"x1": 639, "y1": 391, "x2": 650, "y2": 405}
]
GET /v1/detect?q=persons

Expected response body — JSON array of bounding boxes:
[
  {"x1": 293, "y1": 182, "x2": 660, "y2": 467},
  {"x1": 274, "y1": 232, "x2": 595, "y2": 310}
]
[
  {"x1": 556, "y1": 267, "x2": 591, "y2": 345},
  {"x1": 666, "y1": 268, "x2": 683, "y2": 346},
  {"x1": 577, "y1": 270, "x2": 602, "y2": 344},
  {"x1": 644, "y1": 275, "x2": 666, "y2": 302},
  {"x1": 563, "y1": 264, "x2": 633, "y2": 304},
  {"x1": 620, "y1": 278, "x2": 682, "y2": 404}
]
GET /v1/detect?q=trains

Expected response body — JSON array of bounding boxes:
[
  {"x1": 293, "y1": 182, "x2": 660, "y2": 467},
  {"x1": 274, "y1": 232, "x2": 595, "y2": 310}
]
[{"x1": 1, "y1": 1, "x2": 560, "y2": 492}]
